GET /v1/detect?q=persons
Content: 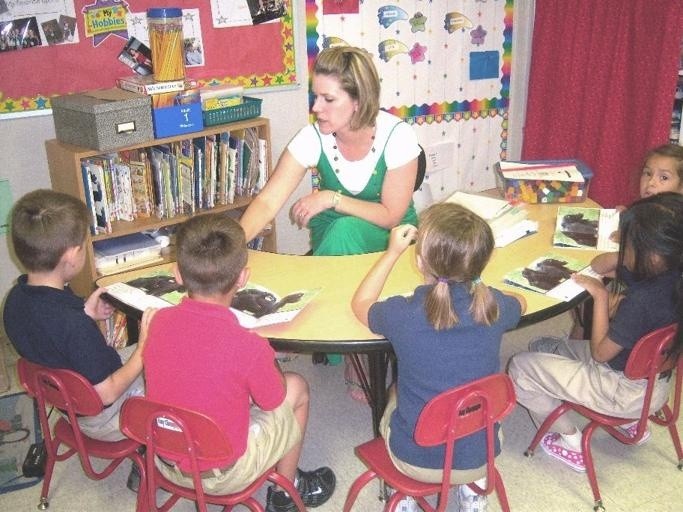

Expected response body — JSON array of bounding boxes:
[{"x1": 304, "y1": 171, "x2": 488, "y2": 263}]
[
  {"x1": 141, "y1": 213, "x2": 337, "y2": 512},
  {"x1": 507, "y1": 192, "x2": 683, "y2": 473},
  {"x1": 351, "y1": 203, "x2": 527, "y2": 512},
  {"x1": 63, "y1": 18, "x2": 71, "y2": 38},
  {"x1": 0, "y1": 27, "x2": 39, "y2": 51},
  {"x1": 3, "y1": 188, "x2": 163, "y2": 492},
  {"x1": 238, "y1": 46, "x2": 419, "y2": 401},
  {"x1": 569, "y1": 143, "x2": 683, "y2": 339},
  {"x1": 187, "y1": 43, "x2": 202, "y2": 65},
  {"x1": 129, "y1": 48, "x2": 152, "y2": 68}
]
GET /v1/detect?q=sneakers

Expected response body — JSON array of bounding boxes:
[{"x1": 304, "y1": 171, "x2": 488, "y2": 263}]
[
  {"x1": 265, "y1": 466, "x2": 337, "y2": 512},
  {"x1": 391, "y1": 488, "x2": 420, "y2": 512},
  {"x1": 537, "y1": 431, "x2": 591, "y2": 473},
  {"x1": 623, "y1": 421, "x2": 653, "y2": 446},
  {"x1": 127, "y1": 450, "x2": 162, "y2": 493},
  {"x1": 455, "y1": 486, "x2": 490, "y2": 512}
]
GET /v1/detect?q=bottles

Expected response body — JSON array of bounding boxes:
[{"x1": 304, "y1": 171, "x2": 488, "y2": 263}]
[{"x1": 144, "y1": 6, "x2": 186, "y2": 83}]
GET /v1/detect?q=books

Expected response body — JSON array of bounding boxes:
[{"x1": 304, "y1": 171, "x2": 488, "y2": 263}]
[
  {"x1": 228, "y1": 283, "x2": 322, "y2": 329},
  {"x1": 81, "y1": 126, "x2": 267, "y2": 311},
  {"x1": 552, "y1": 206, "x2": 621, "y2": 253},
  {"x1": 504, "y1": 252, "x2": 604, "y2": 302},
  {"x1": 446, "y1": 191, "x2": 538, "y2": 249}
]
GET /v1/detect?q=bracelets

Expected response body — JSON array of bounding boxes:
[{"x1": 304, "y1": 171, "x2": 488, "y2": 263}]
[{"x1": 332, "y1": 192, "x2": 341, "y2": 211}]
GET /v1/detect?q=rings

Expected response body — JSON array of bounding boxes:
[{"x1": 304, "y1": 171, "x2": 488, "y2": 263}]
[{"x1": 297, "y1": 212, "x2": 305, "y2": 217}]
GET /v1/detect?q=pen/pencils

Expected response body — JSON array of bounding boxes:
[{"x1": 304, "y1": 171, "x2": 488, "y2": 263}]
[{"x1": 149, "y1": 24, "x2": 186, "y2": 82}]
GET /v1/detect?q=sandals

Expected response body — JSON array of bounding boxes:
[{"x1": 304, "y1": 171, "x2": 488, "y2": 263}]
[{"x1": 344, "y1": 362, "x2": 375, "y2": 401}]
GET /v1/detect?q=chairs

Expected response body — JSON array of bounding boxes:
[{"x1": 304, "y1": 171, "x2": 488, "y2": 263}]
[
  {"x1": 16, "y1": 354, "x2": 144, "y2": 511},
  {"x1": 523, "y1": 323, "x2": 680, "y2": 512},
  {"x1": 111, "y1": 394, "x2": 310, "y2": 511},
  {"x1": 340, "y1": 372, "x2": 517, "y2": 512}
]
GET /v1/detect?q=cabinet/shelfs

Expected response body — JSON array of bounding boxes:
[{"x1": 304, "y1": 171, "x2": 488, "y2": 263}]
[{"x1": 43, "y1": 114, "x2": 275, "y2": 354}]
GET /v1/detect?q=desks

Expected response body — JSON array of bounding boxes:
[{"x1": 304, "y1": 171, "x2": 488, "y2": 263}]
[{"x1": 91, "y1": 188, "x2": 621, "y2": 509}]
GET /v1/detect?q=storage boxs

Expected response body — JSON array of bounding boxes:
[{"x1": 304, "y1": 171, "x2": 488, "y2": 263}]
[
  {"x1": 151, "y1": 102, "x2": 205, "y2": 139},
  {"x1": 50, "y1": 89, "x2": 154, "y2": 150},
  {"x1": 491, "y1": 159, "x2": 593, "y2": 207}
]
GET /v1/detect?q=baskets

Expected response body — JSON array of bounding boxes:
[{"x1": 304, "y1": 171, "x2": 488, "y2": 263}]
[{"x1": 202, "y1": 95, "x2": 262, "y2": 127}]
